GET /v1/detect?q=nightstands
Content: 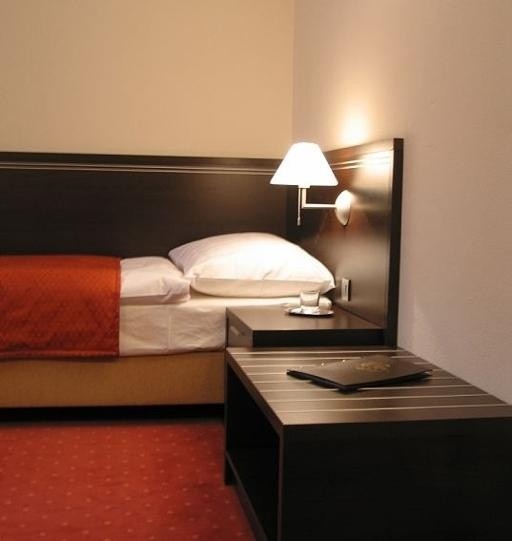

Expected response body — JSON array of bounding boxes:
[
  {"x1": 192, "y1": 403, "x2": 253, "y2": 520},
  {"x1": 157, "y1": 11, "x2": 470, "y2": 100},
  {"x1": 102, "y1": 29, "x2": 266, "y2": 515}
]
[{"x1": 226, "y1": 306, "x2": 384, "y2": 347}]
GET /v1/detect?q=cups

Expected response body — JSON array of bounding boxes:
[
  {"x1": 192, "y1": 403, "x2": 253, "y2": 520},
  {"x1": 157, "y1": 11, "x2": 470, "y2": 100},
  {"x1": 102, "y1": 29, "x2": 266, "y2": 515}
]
[{"x1": 300, "y1": 290, "x2": 320, "y2": 313}]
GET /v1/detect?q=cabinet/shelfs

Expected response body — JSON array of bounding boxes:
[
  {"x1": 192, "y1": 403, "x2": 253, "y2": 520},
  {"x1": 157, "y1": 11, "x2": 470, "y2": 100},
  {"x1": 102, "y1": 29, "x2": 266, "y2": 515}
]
[{"x1": 224, "y1": 345, "x2": 512, "y2": 541}]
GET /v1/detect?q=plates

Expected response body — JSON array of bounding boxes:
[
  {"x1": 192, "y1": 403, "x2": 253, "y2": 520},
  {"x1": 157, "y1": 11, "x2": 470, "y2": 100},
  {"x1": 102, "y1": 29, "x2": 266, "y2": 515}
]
[{"x1": 285, "y1": 308, "x2": 335, "y2": 317}]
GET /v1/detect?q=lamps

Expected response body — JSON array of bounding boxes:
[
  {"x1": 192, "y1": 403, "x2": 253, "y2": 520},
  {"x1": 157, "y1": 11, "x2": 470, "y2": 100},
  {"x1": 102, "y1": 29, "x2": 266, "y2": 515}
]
[{"x1": 269, "y1": 141, "x2": 352, "y2": 227}]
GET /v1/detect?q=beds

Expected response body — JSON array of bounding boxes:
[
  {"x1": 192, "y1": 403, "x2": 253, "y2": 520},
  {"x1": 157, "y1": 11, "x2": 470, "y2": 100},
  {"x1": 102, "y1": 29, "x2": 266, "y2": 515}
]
[{"x1": 0, "y1": 138, "x2": 403, "y2": 408}]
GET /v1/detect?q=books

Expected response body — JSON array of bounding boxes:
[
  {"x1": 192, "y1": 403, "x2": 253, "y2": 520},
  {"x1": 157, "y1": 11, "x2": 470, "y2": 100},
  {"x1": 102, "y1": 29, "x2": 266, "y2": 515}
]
[{"x1": 287, "y1": 354, "x2": 433, "y2": 390}]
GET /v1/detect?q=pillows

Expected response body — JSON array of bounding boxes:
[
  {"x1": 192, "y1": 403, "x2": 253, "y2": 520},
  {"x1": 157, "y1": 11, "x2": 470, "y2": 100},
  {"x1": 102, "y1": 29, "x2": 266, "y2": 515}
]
[{"x1": 168, "y1": 232, "x2": 336, "y2": 297}]
